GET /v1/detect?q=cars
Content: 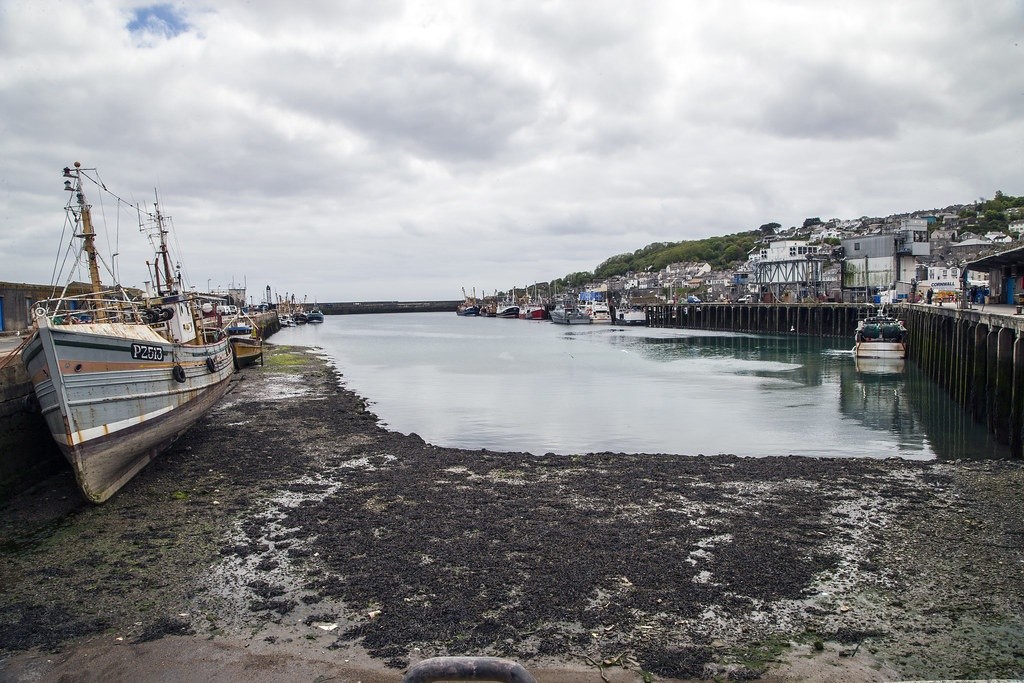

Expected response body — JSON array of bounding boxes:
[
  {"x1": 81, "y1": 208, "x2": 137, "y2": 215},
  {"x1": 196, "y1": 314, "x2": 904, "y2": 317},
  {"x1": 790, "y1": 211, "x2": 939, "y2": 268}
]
[{"x1": 687, "y1": 296, "x2": 701, "y2": 303}]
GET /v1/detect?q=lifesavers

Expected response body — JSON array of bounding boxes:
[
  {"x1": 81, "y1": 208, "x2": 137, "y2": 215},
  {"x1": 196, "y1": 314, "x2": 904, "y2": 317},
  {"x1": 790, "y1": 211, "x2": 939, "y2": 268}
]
[
  {"x1": 172, "y1": 365, "x2": 187, "y2": 383},
  {"x1": 205, "y1": 357, "x2": 217, "y2": 374}
]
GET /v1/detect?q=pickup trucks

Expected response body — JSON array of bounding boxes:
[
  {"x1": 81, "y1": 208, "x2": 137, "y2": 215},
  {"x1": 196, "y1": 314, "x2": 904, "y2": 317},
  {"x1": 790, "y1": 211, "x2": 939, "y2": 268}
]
[{"x1": 737, "y1": 295, "x2": 760, "y2": 303}]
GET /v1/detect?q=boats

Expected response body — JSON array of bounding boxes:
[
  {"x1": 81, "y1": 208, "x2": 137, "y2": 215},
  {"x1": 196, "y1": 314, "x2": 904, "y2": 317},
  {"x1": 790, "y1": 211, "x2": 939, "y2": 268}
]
[{"x1": 855, "y1": 282, "x2": 908, "y2": 357}]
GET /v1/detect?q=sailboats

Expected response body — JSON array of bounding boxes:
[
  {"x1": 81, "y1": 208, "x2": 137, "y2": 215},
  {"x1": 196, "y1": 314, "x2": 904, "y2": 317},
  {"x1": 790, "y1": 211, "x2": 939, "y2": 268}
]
[
  {"x1": 456, "y1": 279, "x2": 647, "y2": 325},
  {"x1": 21, "y1": 162, "x2": 327, "y2": 504}
]
